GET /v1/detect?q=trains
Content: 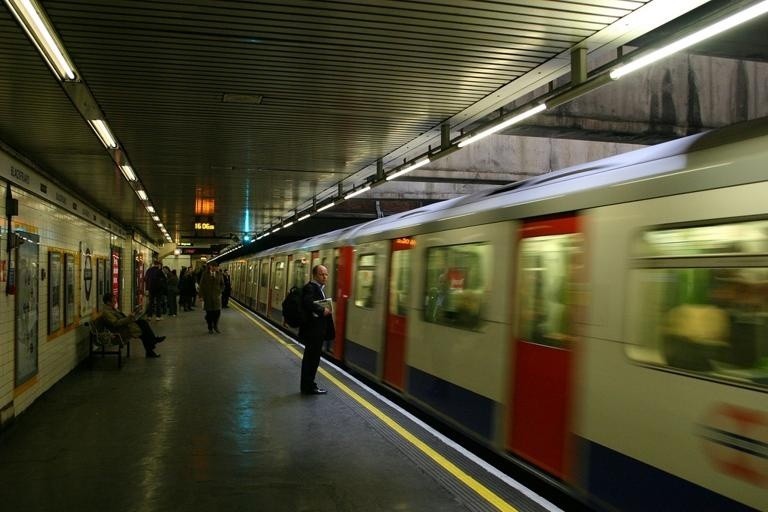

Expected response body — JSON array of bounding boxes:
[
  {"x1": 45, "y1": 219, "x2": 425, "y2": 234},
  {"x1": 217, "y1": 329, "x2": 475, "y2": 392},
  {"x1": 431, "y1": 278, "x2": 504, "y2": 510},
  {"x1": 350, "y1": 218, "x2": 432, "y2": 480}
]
[{"x1": 206, "y1": 112, "x2": 768, "y2": 512}]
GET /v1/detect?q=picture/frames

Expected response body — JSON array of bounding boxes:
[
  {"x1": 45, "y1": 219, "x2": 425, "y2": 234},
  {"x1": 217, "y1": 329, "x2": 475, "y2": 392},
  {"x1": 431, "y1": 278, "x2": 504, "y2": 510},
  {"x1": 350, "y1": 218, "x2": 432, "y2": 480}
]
[
  {"x1": 48, "y1": 251, "x2": 62, "y2": 336},
  {"x1": 14, "y1": 230, "x2": 40, "y2": 389},
  {"x1": 65, "y1": 252, "x2": 75, "y2": 327},
  {"x1": 96, "y1": 255, "x2": 105, "y2": 311},
  {"x1": 105, "y1": 259, "x2": 112, "y2": 300}
]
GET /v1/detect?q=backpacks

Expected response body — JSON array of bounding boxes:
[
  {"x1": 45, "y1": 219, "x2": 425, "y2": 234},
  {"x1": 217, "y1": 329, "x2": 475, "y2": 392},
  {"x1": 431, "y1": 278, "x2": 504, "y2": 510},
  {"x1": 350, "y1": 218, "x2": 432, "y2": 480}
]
[{"x1": 282, "y1": 285, "x2": 306, "y2": 329}]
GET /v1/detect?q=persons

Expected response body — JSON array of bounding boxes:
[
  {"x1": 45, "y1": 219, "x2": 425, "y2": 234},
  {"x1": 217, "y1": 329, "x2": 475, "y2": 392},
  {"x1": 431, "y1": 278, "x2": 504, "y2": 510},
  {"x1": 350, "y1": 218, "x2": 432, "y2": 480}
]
[
  {"x1": 103, "y1": 293, "x2": 166, "y2": 357},
  {"x1": 426, "y1": 273, "x2": 453, "y2": 324},
  {"x1": 299, "y1": 265, "x2": 337, "y2": 395},
  {"x1": 144, "y1": 260, "x2": 232, "y2": 334}
]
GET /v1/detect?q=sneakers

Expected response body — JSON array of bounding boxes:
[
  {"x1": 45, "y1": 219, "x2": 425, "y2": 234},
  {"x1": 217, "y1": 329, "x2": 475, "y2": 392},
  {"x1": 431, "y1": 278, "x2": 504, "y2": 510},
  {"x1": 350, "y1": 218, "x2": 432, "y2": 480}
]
[
  {"x1": 208, "y1": 328, "x2": 221, "y2": 334},
  {"x1": 180, "y1": 305, "x2": 195, "y2": 313},
  {"x1": 147, "y1": 313, "x2": 176, "y2": 321}
]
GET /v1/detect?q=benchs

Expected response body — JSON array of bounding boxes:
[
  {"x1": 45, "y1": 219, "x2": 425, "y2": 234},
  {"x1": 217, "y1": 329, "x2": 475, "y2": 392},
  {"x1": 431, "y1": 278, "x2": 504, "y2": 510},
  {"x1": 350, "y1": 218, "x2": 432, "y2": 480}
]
[{"x1": 84, "y1": 317, "x2": 131, "y2": 367}]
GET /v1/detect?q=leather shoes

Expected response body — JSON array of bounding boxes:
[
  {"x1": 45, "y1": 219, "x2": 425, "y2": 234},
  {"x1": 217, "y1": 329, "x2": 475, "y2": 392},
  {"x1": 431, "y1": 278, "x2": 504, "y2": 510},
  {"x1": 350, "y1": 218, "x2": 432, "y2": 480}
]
[
  {"x1": 155, "y1": 336, "x2": 166, "y2": 344},
  {"x1": 145, "y1": 350, "x2": 161, "y2": 359},
  {"x1": 300, "y1": 386, "x2": 328, "y2": 395}
]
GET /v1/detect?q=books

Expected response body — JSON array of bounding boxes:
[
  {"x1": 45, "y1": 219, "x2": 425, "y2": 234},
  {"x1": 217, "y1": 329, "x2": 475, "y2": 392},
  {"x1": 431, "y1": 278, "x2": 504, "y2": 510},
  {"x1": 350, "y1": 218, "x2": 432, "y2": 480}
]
[{"x1": 313, "y1": 298, "x2": 334, "y2": 316}]
